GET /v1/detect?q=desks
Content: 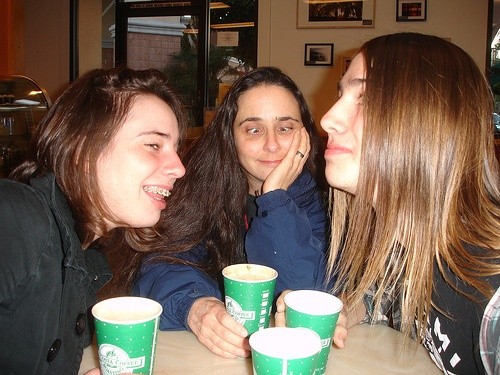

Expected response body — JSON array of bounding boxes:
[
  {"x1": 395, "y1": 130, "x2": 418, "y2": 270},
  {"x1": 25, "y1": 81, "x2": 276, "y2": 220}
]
[{"x1": 80, "y1": 314, "x2": 442, "y2": 375}]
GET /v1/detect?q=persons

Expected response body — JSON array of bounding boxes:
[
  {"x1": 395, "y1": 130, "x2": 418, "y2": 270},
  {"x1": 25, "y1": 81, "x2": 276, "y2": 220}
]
[
  {"x1": 275, "y1": 31, "x2": 500, "y2": 375},
  {"x1": 0, "y1": 65, "x2": 186, "y2": 375},
  {"x1": 132, "y1": 66, "x2": 332, "y2": 359}
]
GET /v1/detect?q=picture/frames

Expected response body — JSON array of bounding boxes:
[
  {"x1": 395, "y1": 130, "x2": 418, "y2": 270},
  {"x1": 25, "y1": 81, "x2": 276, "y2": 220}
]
[
  {"x1": 297, "y1": 0, "x2": 377, "y2": 29},
  {"x1": 305, "y1": 43, "x2": 334, "y2": 66},
  {"x1": 395, "y1": 0, "x2": 427, "y2": 22},
  {"x1": 341, "y1": 57, "x2": 352, "y2": 75}
]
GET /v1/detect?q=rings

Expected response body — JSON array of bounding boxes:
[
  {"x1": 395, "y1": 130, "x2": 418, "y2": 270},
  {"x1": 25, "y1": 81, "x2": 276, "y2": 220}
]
[{"x1": 296, "y1": 151, "x2": 305, "y2": 158}]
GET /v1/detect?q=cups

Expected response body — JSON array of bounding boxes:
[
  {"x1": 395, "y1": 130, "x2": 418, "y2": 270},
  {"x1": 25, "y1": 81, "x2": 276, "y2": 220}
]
[
  {"x1": 222, "y1": 263, "x2": 278, "y2": 338},
  {"x1": 284, "y1": 289, "x2": 343, "y2": 375},
  {"x1": 248, "y1": 327, "x2": 322, "y2": 375},
  {"x1": 91, "y1": 296, "x2": 163, "y2": 374}
]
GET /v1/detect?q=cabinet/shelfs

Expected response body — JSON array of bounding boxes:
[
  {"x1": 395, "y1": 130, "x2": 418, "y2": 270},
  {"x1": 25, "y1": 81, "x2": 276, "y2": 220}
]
[{"x1": 199, "y1": 1, "x2": 258, "y2": 115}]
